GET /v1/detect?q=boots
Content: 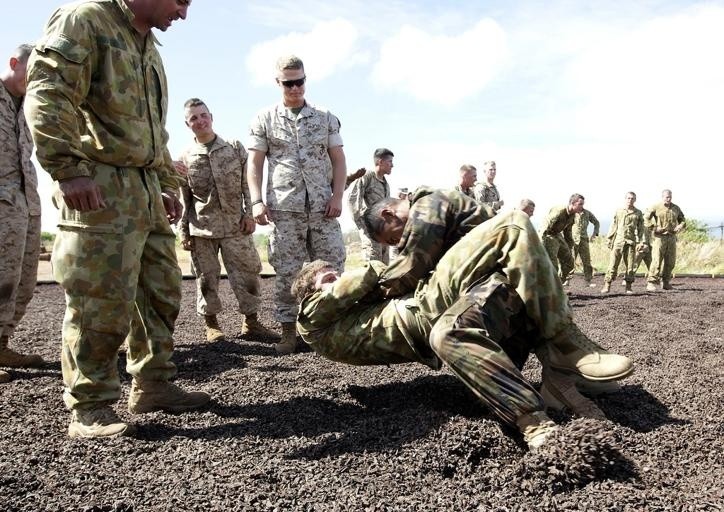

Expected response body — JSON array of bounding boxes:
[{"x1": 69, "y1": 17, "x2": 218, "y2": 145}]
[
  {"x1": 517, "y1": 323, "x2": 635, "y2": 450},
  {"x1": 272, "y1": 321, "x2": 296, "y2": 353},
  {"x1": 647, "y1": 281, "x2": 671, "y2": 292},
  {"x1": 601, "y1": 282, "x2": 610, "y2": 294},
  {"x1": 622, "y1": 280, "x2": 632, "y2": 293},
  {"x1": 204, "y1": 313, "x2": 225, "y2": 343},
  {"x1": 564, "y1": 280, "x2": 595, "y2": 288},
  {"x1": 242, "y1": 313, "x2": 280, "y2": 339},
  {"x1": 67, "y1": 408, "x2": 136, "y2": 439},
  {"x1": 129, "y1": 378, "x2": 211, "y2": 414},
  {"x1": 1, "y1": 337, "x2": 44, "y2": 383}
]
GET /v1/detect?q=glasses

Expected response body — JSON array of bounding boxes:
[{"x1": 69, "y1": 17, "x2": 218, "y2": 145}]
[{"x1": 281, "y1": 76, "x2": 305, "y2": 88}]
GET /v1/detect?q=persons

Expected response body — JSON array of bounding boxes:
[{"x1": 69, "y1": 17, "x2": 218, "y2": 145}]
[
  {"x1": 360, "y1": 186, "x2": 609, "y2": 452},
  {"x1": 478, "y1": 160, "x2": 505, "y2": 216},
  {"x1": 329, "y1": 167, "x2": 366, "y2": 192},
  {"x1": 539, "y1": 193, "x2": 585, "y2": 286},
  {"x1": 171, "y1": 96, "x2": 285, "y2": 348},
  {"x1": 246, "y1": 56, "x2": 351, "y2": 354},
  {"x1": 621, "y1": 210, "x2": 655, "y2": 286},
  {"x1": 289, "y1": 209, "x2": 636, "y2": 396},
  {"x1": 346, "y1": 147, "x2": 393, "y2": 267},
  {"x1": 601, "y1": 192, "x2": 644, "y2": 294},
  {"x1": 0, "y1": 43, "x2": 51, "y2": 383},
  {"x1": 454, "y1": 164, "x2": 478, "y2": 204},
  {"x1": 19, "y1": 0, "x2": 212, "y2": 439},
  {"x1": 643, "y1": 188, "x2": 687, "y2": 292},
  {"x1": 563, "y1": 206, "x2": 600, "y2": 288},
  {"x1": 512, "y1": 198, "x2": 535, "y2": 220}
]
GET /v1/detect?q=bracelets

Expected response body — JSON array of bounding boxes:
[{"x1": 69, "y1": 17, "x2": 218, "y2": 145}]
[{"x1": 250, "y1": 199, "x2": 263, "y2": 207}]
